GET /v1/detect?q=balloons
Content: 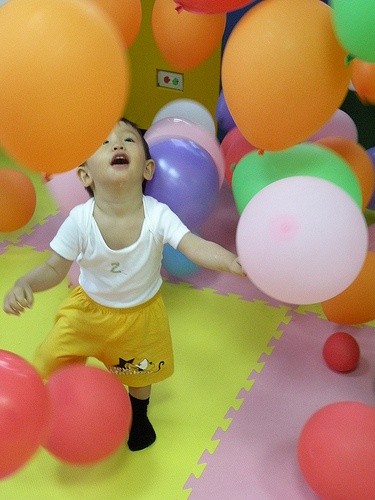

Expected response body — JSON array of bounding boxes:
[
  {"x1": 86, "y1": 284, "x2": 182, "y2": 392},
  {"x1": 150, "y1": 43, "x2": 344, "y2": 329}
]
[
  {"x1": 298, "y1": 403, "x2": 375, "y2": 500},
  {"x1": 0, "y1": 349, "x2": 131, "y2": 479},
  {"x1": 0, "y1": 0, "x2": 375, "y2": 372}
]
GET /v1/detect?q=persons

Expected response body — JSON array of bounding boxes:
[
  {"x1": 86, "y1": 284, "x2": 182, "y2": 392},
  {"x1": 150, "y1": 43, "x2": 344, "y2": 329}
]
[{"x1": 3, "y1": 117, "x2": 247, "y2": 451}]
